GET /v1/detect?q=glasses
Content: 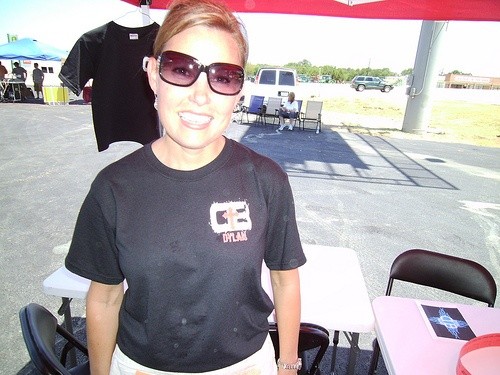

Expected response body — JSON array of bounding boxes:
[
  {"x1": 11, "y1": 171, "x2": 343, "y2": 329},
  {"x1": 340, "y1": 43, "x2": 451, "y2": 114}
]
[{"x1": 157, "y1": 50, "x2": 245, "y2": 95}]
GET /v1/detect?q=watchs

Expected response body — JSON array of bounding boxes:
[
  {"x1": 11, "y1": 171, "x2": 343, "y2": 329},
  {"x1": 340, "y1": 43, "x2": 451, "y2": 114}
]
[{"x1": 277, "y1": 357, "x2": 302, "y2": 370}]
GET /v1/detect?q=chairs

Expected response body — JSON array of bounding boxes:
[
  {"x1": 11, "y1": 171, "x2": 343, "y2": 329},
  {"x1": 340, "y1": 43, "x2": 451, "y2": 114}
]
[
  {"x1": 232, "y1": 95, "x2": 323, "y2": 133},
  {"x1": 17, "y1": 247, "x2": 496, "y2": 375}
]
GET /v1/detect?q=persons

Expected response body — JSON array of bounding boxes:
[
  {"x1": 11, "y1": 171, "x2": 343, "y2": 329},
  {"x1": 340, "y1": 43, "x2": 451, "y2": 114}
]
[
  {"x1": 64, "y1": 0, "x2": 306, "y2": 375},
  {"x1": 279, "y1": 92, "x2": 298, "y2": 131},
  {"x1": 0, "y1": 61, "x2": 45, "y2": 100}
]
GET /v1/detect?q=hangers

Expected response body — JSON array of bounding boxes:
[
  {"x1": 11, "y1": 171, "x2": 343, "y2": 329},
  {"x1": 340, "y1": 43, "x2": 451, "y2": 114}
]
[{"x1": 113, "y1": 0, "x2": 154, "y2": 22}]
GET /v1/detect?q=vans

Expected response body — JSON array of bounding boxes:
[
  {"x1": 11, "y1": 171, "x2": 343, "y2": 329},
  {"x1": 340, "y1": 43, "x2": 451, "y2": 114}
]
[{"x1": 252, "y1": 67, "x2": 300, "y2": 99}]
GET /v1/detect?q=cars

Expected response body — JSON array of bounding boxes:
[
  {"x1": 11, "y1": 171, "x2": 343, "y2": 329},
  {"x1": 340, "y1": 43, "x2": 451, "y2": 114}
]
[{"x1": 350, "y1": 75, "x2": 395, "y2": 93}]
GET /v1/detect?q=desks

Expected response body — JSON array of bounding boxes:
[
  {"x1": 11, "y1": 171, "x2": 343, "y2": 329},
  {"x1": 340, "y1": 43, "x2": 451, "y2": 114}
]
[
  {"x1": 371, "y1": 297, "x2": 500, "y2": 375},
  {"x1": 42, "y1": 242, "x2": 373, "y2": 375},
  {"x1": 0, "y1": 81, "x2": 25, "y2": 103}
]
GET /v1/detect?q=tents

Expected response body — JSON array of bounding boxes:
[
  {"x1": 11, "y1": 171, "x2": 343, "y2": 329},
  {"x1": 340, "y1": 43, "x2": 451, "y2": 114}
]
[{"x1": 0, "y1": 38, "x2": 73, "y2": 105}]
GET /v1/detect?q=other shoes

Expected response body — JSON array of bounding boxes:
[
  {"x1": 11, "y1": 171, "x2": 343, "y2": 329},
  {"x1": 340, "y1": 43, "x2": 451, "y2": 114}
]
[
  {"x1": 279, "y1": 125, "x2": 285, "y2": 131},
  {"x1": 287, "y1": 124, "x2": 293, "y2": 131}
]
